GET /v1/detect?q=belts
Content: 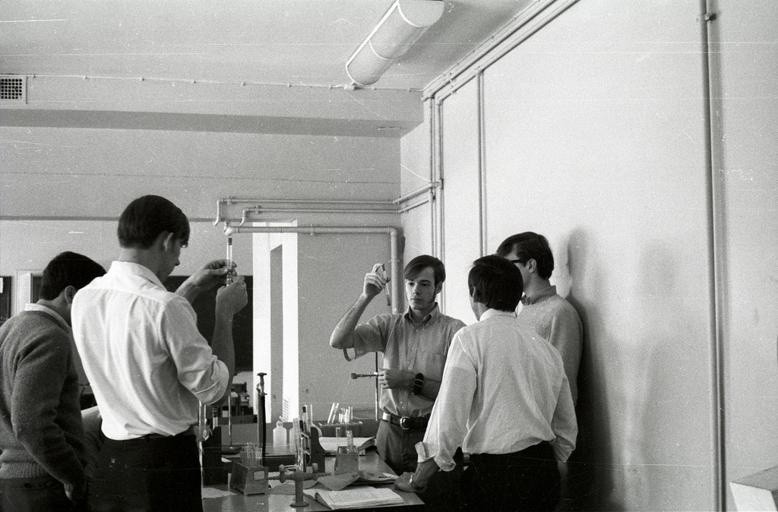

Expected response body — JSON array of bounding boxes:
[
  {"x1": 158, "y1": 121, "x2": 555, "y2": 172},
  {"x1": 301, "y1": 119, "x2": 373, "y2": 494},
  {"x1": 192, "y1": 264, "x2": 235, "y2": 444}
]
[{"x1": 380, "y1": 411, "x2": 429, "y2": 429}]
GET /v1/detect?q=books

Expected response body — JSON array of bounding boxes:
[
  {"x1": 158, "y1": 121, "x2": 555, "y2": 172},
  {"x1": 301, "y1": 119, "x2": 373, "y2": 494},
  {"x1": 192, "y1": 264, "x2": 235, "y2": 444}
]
[
  {"x1": 302, "y1": 486, "x2": 404, "y2": 509},
  {"x1": 319, "y1": 436, "x2": 376, "y2": 455}
]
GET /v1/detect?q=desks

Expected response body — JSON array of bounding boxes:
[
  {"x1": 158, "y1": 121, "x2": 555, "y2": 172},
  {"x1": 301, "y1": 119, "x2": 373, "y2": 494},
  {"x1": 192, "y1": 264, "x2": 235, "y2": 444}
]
[{"x1": 198, "y1": 442, "x2": 425, "y2": 512}]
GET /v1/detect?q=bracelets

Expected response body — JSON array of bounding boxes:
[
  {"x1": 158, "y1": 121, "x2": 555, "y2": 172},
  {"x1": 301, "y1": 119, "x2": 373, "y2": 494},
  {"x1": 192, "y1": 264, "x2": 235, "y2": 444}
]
[{"x1": 408, "y1": 471, "x2": 426, "y2": 491}]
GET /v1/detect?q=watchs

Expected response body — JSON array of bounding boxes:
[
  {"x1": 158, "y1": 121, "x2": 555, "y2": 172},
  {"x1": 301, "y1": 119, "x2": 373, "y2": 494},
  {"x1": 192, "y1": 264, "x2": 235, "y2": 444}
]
[{"x1": 413, "y1": 373, "x2": 424, "y2": 395}]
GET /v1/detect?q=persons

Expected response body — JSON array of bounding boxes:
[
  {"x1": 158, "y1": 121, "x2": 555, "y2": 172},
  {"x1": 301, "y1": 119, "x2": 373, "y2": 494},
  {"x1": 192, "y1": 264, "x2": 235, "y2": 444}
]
[
  {"x1": 496, "y1": 231, "x2": 584, "y2": 510},
  {"x1": 396, "y1": 257, "x2": 578, "y2": 510},
  {"x1": 328, "y1": 254, "x2": 469, "y2": 509},
  {"x1": 72, "y1": 194, "x2": 247, "y2": 511},
  {"x1": 0, "y1": 251, "x2": 107, "y2": 510}
]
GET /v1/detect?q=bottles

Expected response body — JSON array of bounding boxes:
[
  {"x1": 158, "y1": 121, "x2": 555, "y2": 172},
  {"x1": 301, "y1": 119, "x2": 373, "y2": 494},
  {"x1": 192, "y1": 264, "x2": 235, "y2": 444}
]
[
  {"x1": 290, "y1": 418, "x2": 301, "y2": 447},
  {"x1": 273, "y1": 421, "x2": 287, "y2": 444},
  {"x1": 381, "y1": 264, "x2": 392, "y2": 305},
  {"x1": 304, "y1": 403, "x2": 354, "y2": 448},
  {"x1": 226, "y1": 237, "x2": 233, "y2": 285},
  {"x1": 240, "y1": 444, "x2": 264, "y2": 465}
]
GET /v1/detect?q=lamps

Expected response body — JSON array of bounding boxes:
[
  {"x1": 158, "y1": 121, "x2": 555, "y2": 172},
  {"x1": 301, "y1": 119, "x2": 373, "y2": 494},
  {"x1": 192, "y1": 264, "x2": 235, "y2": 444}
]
[{"x1": 344, "y1": 0, "x2": 446, "y2": 86}]
